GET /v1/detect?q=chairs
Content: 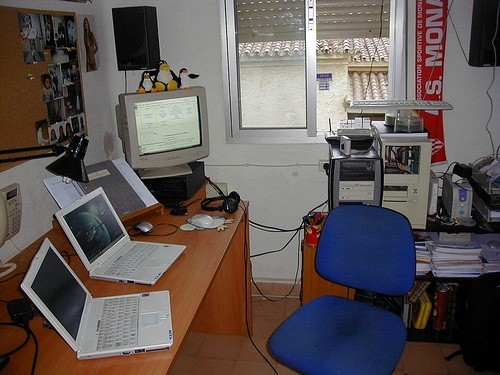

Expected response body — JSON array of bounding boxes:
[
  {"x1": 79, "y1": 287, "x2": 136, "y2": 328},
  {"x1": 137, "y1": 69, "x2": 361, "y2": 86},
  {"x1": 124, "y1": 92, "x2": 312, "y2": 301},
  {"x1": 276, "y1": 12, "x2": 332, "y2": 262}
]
[{"x1": 266, "y1": 203, "x2": 416, "y2": 375}]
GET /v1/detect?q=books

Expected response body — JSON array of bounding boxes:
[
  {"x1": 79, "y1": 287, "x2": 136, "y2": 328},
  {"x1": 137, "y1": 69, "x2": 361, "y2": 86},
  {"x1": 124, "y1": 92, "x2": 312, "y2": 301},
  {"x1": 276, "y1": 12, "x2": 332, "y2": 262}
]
[
  {"x1": 72, "y1": 160, "x2": 147, "y2": 216},
  {"x1": 402, "y1": 294, "x2": 413, "y2": 329},
  {"x1": 414, "y1": 231, "x2": 500, "y2": 278}
]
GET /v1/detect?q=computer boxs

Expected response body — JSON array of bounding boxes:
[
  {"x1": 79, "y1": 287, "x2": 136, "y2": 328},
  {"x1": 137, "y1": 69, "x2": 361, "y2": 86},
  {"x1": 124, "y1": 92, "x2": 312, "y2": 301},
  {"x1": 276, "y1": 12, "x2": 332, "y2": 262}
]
[
  {"x1": 328, "y1": 143, "x2": 384, "y2": 211},
  {"x1": 134, "y1": 162, "x2": 205, "y2": 201}
]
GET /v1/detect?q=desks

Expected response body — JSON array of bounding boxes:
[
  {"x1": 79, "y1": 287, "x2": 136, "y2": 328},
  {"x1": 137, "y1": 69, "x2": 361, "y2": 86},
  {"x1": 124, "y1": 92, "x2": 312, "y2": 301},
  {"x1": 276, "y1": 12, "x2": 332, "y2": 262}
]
[{"x1": 0, "y1": 196, "x2": 252, "y2": 375}]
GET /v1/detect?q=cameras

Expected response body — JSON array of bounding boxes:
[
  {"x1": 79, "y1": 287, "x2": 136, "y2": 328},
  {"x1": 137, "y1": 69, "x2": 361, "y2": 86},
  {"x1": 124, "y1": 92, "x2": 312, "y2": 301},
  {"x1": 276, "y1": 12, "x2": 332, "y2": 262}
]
[{"x1": 339, "y1": 135, "x2": 352, "y2": 156}]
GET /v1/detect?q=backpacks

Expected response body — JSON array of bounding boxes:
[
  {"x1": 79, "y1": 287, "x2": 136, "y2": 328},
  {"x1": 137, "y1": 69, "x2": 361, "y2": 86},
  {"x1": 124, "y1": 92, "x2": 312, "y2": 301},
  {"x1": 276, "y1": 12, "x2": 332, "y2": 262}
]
[{"x1": 444, "y1": 272, "x2": 500, "y2": 372}]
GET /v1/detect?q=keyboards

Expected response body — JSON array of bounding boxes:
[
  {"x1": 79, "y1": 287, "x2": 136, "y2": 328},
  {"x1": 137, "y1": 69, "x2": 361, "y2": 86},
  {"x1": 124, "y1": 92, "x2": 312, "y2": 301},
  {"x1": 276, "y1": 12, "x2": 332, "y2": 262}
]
[{"x1": 347, "y1": 99, "x2": 453, "y2": 113}]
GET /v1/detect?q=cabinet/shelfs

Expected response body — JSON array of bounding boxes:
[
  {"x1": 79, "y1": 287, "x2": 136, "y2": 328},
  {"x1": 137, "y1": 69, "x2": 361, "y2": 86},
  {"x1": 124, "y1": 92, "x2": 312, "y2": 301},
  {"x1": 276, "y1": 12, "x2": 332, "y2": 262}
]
[
  {"x1": 397, "y1": 227, "x2": 500, "y2": 343},
  {"x1": 299, "y1": 237, "x2": 356, "y2": 307}
]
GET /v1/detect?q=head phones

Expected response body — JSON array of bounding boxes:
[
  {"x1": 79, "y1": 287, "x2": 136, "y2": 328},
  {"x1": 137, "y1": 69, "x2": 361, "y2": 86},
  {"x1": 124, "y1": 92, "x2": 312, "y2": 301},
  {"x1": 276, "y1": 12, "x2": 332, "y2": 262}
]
[{"x1": 201, "y1": 192, "x2": 241, "y2": 214}]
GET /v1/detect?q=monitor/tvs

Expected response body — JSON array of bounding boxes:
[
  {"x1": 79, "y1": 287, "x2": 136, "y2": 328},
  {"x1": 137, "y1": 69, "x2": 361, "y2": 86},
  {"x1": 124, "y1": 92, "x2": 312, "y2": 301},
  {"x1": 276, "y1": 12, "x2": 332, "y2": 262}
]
[{"x1": 115, "y1": 86, "x2": 210, "y2": 180}]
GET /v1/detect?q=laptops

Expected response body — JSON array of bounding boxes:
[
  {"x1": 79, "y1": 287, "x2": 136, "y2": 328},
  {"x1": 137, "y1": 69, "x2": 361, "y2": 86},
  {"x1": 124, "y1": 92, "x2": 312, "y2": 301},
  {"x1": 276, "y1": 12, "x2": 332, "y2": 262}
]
[
  {"x1": 19, "y1": 236, "x2": 173, "y2": 360},
  {"x1": 54, "y1": 186, "x2": 187, "y2": 287}
]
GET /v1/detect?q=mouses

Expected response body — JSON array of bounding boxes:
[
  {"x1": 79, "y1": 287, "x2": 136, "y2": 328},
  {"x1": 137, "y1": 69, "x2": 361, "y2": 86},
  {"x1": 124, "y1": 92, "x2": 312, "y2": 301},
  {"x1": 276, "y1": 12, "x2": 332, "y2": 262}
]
[
  {"x1": 169, "y1": 206, "x2": 187, "y2": 216},
  {"x1": 135, "y1": 221, "x2": 154, "y2": 232}
]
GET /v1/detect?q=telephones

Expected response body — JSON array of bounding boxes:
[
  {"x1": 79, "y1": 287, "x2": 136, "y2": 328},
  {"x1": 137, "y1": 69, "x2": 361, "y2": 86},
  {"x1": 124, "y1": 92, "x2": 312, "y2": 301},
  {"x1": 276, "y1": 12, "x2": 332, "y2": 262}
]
[{"x1": 0, "y1": 183, "x2": 22, "y2": 251}]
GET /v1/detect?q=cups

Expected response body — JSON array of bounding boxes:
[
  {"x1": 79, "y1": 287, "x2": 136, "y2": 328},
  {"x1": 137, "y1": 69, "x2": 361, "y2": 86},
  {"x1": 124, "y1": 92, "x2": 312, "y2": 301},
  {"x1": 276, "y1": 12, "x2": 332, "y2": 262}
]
[{"x1": 304, "y1": 224, "x2": 323, "y2": 246}]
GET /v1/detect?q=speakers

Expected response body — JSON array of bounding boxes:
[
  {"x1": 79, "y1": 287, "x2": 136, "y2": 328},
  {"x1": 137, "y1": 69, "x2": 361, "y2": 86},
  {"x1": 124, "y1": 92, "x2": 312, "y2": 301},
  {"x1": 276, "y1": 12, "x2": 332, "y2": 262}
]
[
  {"x1": 112, "y1": 6, "x2": 160, "y2": 72},
  {"x1": 467, "y1": 0, "x2": 500, "y2": 68}
]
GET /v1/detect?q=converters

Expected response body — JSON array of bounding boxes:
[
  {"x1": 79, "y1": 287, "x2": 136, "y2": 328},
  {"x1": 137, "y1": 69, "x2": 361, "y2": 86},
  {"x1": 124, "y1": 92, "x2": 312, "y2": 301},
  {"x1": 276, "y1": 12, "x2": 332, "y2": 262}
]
[
  {"x1": 7, "y1": 295, "x2": 34, "y2": 323},
  {"x1": 454, "y1": 164, "x2": 473, "y2": 179}
]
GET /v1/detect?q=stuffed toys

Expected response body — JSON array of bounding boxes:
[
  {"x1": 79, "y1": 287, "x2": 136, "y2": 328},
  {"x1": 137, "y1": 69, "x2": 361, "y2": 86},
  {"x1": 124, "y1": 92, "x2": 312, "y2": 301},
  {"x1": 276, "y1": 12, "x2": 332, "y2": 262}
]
[
  {"x1": 149, "y1": 59, "x2": 178, "y2": 92},
  {"x1": 137, "y1": 71, "x2": 158, "y2": 93},
  {"x1": 178, "y1": 68, "x2": 200, "y2": 90}
]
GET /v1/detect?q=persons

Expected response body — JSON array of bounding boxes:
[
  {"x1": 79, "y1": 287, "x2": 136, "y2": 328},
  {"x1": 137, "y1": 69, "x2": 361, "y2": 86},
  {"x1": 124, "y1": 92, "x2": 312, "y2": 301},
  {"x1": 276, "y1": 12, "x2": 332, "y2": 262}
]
[
  {"x1": 50, "y1": 129, "x2": 58, "y2": 145},
  {"x1": 63, "y1": 72, "x2": 71, "y2": 84},
  {"x1": 65, "y1": 122, "x2": 73, "y2": 137},
  {"x1": 83, "y1": 18, "x2": 99, "y2": 73},
  {"x1": 52, "y1": 71, "x2": 59, "y2": 98},
  {"x1": 80, "y1": 117, "x2": 85, "y2": 132},
  {"x1": 37, "y1": 124, "x2": 44, "y2": 145},
  {"x1": 26, "y1": 45, "x2": 44, "y2": 64},
  {"x1": 70, "y1": 62, "x2": 80, "y2": 81},
  {"x1": 40, "y1": 74, "x2": 54, "y2": 102},
  {"x1": 58, "y1": 125, "x2": 65, "y2": 140}
]
